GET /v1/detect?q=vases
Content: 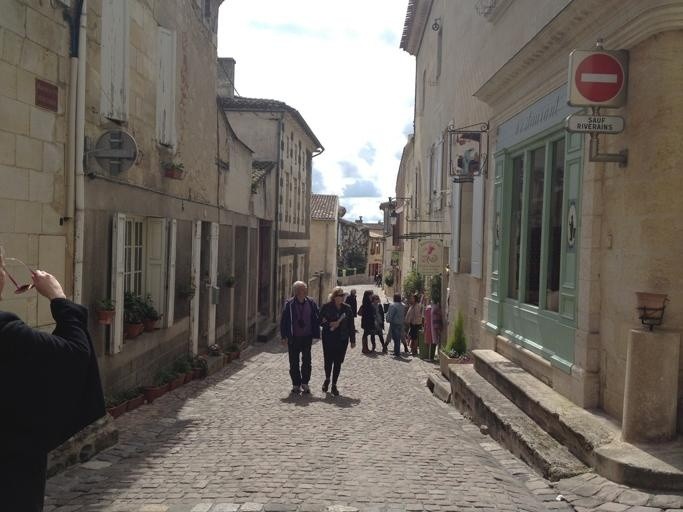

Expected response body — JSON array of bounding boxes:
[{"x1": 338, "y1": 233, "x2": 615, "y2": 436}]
[
  {"x1": 212, "y1": 350, "x2": 218, "y2": 356},
  {"x1": 191, "y1": 368, "x2": 202, "y2": 378}
]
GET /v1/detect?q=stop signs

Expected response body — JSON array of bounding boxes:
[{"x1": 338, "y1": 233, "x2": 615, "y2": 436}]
[{"x1": 568, "y1": 48, "x2": 626, "y2": 107}]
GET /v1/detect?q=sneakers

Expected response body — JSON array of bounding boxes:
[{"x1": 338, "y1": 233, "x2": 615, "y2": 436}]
[{"x1": 293, "y1": 380, "x2": 339, "y2": 396}]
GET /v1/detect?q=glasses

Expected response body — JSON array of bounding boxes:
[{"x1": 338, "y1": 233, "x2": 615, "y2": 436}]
[
  {"x1": 337, "y1": 294, "x2": 345, "y2": 297},
  {"x1": 3, "y1": 258, "x2": 37, "y2": 294}
]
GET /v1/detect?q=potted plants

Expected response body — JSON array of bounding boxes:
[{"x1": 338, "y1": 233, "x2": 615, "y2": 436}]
[
  {"x1": 164, "y1": 161, "x2": 185, "y2": 179},
  {"x1": 95, "y1": 287, "x2": 164, "y2": 339},
  {"x1": 438, "y1": 312, "x2": 473, "y2": 381},
  {"x1": 383, "y1": 270, "x2": 395, "y2": 297},
  {"x1": 222, "y1": 273, "x2": 238, "y2": 288},
  {"x1": 104, "y1": 353, "x2": 193, "y2": 418},
  {"x1": 223, "y1": 345, "x2": 240, "y2": 365}
]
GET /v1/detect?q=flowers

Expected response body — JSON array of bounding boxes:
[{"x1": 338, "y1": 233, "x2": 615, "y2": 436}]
[
  {"x1": 190, "y1": 351, "x2": 208, "y2": 368},
  {"x1": 208, "y1": 343, "x2": 222, "y2": 351}
]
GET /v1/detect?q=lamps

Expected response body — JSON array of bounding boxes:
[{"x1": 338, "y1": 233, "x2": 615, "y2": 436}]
[
  {"x1": 387, "y1": 196, "x2": 413, "y2": 226},
  {"x1": 449, "y1": 121, "x2": 489, "y2": 183},
  {"x1": 633, "y1": 290, "x2": 668, "y2": 331}
]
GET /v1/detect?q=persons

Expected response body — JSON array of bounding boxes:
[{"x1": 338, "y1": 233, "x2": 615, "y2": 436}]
[
  {"x1": 0, "y1": 247, "x2": 106, "y2": 512},
  {"x1": 345, "y1": 290, "x2": 441, "y2": 362},
  {"x1": 280, "y1": 281, "x2": 327, "y2": 392},
  {"x1": 319, "y1": 287, "x2": 355, "y2": 396}
]
